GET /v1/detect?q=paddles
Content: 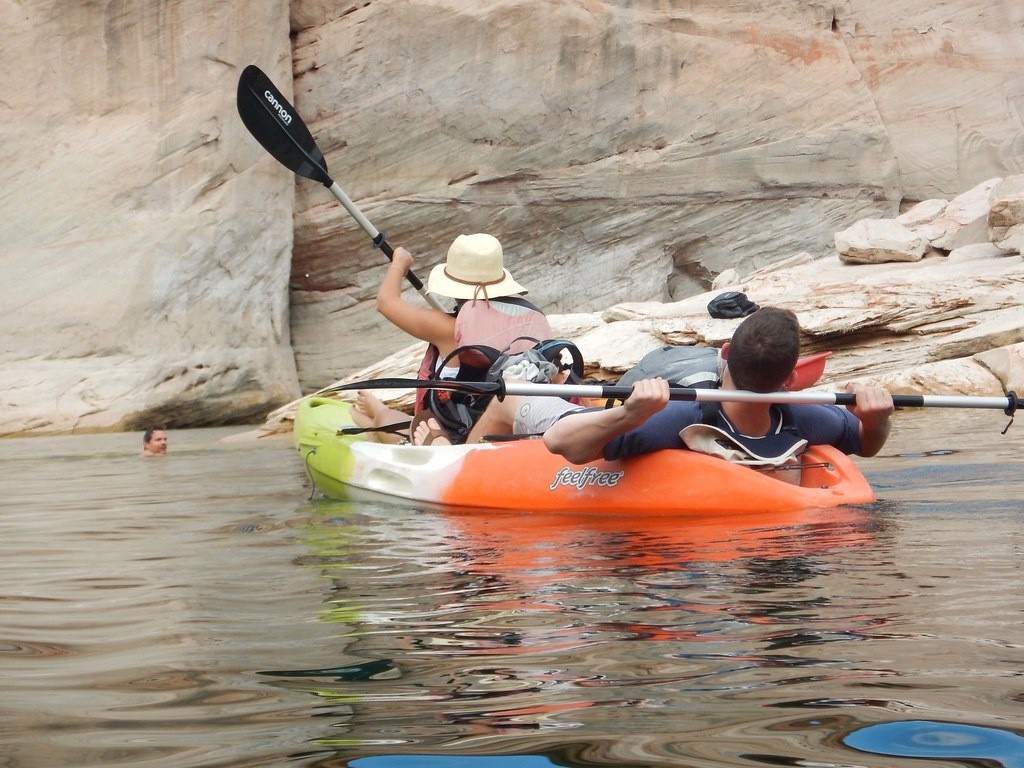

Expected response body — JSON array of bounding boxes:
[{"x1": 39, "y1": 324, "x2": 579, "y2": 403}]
[
  {"x1": 233, "y1": 62, "x2": 450, "y2": 315},
  {"x1": 316, "y1": 374, "x2": 1024, "y2": 418}
]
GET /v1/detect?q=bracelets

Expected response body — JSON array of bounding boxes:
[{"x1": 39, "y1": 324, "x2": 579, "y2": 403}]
[{"x1": 387, "y1": 264, "x2": 405, "y2": 276}]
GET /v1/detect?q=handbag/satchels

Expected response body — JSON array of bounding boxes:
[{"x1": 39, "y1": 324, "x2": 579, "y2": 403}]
[{"x1": 707, "y1": 292, "x2": 760, "y2": 318}]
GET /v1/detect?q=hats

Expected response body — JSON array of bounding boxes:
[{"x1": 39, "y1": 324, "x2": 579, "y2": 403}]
[{"x1": 428, "y1": 232, "x2": 529, "y2": 309}]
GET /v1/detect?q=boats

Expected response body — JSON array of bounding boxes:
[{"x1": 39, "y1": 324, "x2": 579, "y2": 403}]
[{"x1": 293, "y1": 393, "x2": 875, "y2": 514}]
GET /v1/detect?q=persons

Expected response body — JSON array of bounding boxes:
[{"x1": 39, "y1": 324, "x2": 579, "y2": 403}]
[
  {"x1": 347, "y1": 232, "x2": 548, "y2": 440},
  {"x1": 408, "y1": 307, "x2": 895, "y2": 480}
]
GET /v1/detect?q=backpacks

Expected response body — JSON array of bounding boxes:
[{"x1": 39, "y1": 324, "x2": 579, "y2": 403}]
[{"x1": 429, "y1": 336, "x2": 584, "y2": 445}]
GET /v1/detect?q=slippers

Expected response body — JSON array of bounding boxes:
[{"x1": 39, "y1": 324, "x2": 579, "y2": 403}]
[{"x1": 409, "y1": 410, "x2": 454, "y2": 446}]
